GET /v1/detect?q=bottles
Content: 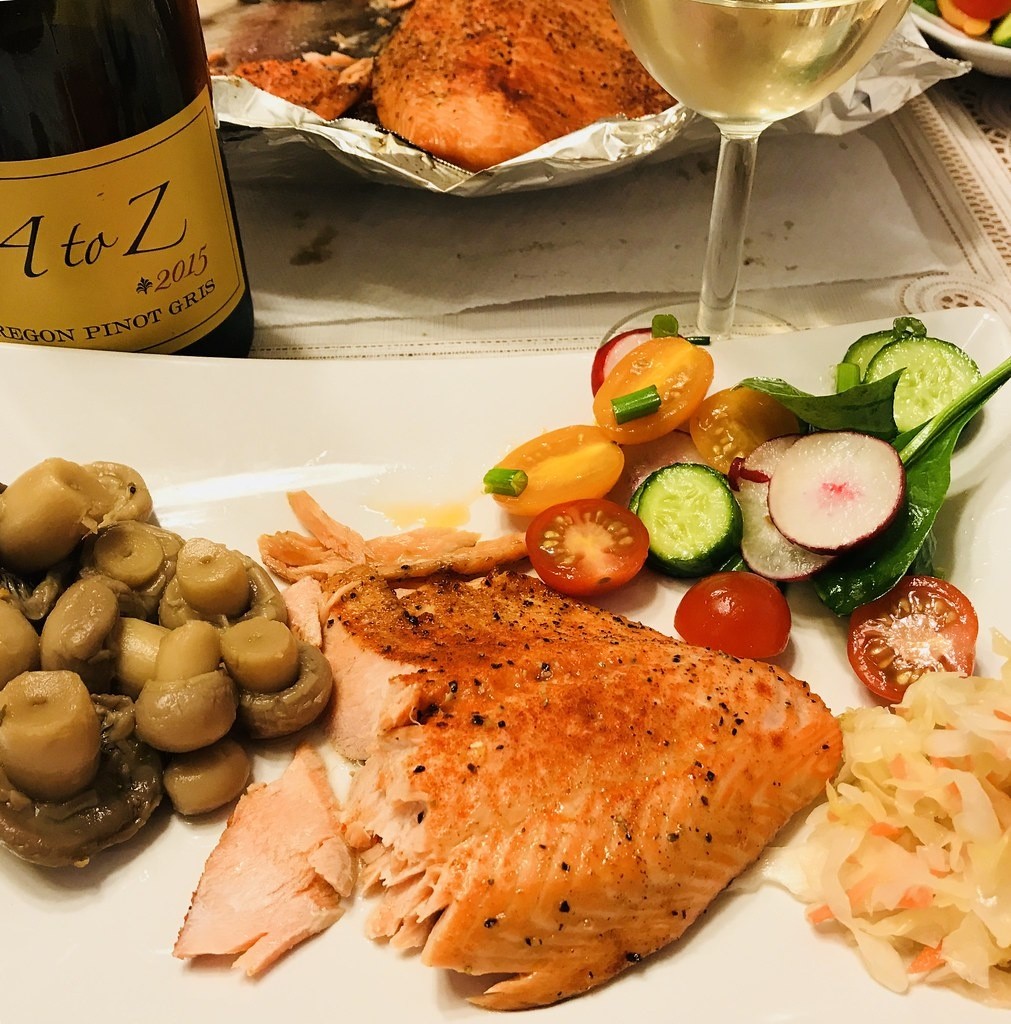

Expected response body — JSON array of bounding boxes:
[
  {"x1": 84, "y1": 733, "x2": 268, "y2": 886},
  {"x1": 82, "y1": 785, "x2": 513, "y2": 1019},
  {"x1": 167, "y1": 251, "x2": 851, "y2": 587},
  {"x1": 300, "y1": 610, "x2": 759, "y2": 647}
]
[{"x1": 0, "y1": 1, "x2": 258, "y2": 367}]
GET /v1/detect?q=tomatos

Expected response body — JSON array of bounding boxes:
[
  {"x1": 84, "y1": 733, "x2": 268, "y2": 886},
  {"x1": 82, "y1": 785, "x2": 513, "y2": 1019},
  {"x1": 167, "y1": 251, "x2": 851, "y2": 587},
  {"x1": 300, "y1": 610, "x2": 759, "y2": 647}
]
[{"x1": 478, "y1": 339, "x2": 979, "y2": 700}]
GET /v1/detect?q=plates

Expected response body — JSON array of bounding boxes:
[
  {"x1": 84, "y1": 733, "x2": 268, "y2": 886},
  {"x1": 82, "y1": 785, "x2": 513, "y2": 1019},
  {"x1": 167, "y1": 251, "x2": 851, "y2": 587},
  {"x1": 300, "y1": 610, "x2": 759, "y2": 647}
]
[
  {"x1": 906, "y1": 0, "x2": 1011, "y2": 80},
  {"x1": 2, "y1": 304, "x2": 1011, "y2": 1022}
]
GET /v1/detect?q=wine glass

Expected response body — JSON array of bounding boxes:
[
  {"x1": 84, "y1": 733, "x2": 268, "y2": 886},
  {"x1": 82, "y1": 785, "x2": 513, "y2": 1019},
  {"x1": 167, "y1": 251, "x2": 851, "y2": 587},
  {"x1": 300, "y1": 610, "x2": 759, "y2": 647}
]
[{"x1": 605, "y1": 0, "x2": 913, "y2": 366}]
